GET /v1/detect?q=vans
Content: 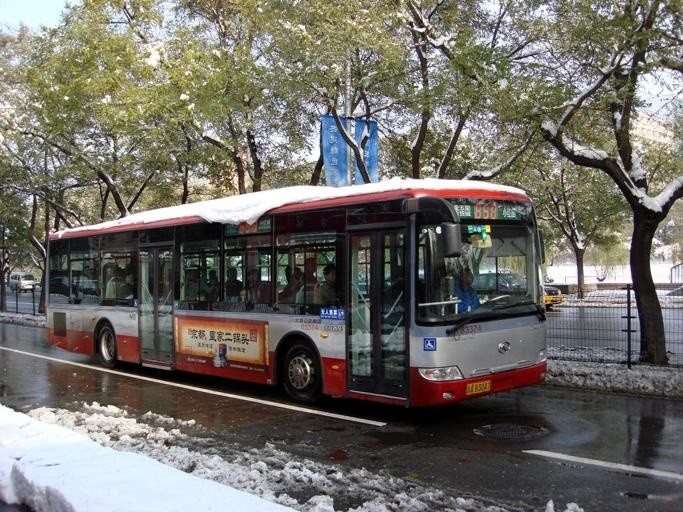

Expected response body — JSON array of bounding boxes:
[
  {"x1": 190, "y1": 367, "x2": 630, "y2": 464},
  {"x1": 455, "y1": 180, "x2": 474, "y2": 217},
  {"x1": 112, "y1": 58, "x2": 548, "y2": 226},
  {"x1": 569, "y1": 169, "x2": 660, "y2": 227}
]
[{"x1": 9, "y1": 271, "x2": 35, "y2": 293}]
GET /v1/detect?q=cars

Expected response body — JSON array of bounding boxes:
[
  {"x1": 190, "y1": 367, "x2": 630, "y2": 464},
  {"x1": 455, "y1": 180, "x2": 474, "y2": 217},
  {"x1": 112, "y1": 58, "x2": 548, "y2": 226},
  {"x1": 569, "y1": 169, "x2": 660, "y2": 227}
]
[{"x1": 542, "y1": 284, "x2": 563, "y2": 309}]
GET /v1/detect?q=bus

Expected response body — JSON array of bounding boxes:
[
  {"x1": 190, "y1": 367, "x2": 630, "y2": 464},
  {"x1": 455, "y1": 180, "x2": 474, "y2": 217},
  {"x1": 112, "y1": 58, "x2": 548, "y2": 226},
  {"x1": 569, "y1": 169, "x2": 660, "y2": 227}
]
[{"x1": 42, "y1": 174, "x2": 549, "y2": 409}]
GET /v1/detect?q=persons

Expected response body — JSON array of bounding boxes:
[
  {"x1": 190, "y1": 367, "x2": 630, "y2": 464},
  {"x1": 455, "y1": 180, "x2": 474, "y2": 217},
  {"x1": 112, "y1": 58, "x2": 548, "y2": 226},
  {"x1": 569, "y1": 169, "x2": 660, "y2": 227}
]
[
  {"x1": 237, "y1": 267, "x2": 265, "y2": 306},
  {"x1": 275, "y1": 265, "x2": 303, "y2": 301},
  {"x1": 224, "y1": 268, "x2": 244, "y2": 297},
  {"x1": 184, "y1": 261, "x2": 221, "y2": 304},
  {"x1": 319, "y1": 264, "x2": 337, "y2": 306},
  {"x1": 300, "y1": 257, "x2": 320, "y2": 286},
  {"x1": 452, "y1": 266, "x2": 487, "y2": 314},
  {"x1": 76, "y1": 260, "x2": 135, "y2": 302}
]
